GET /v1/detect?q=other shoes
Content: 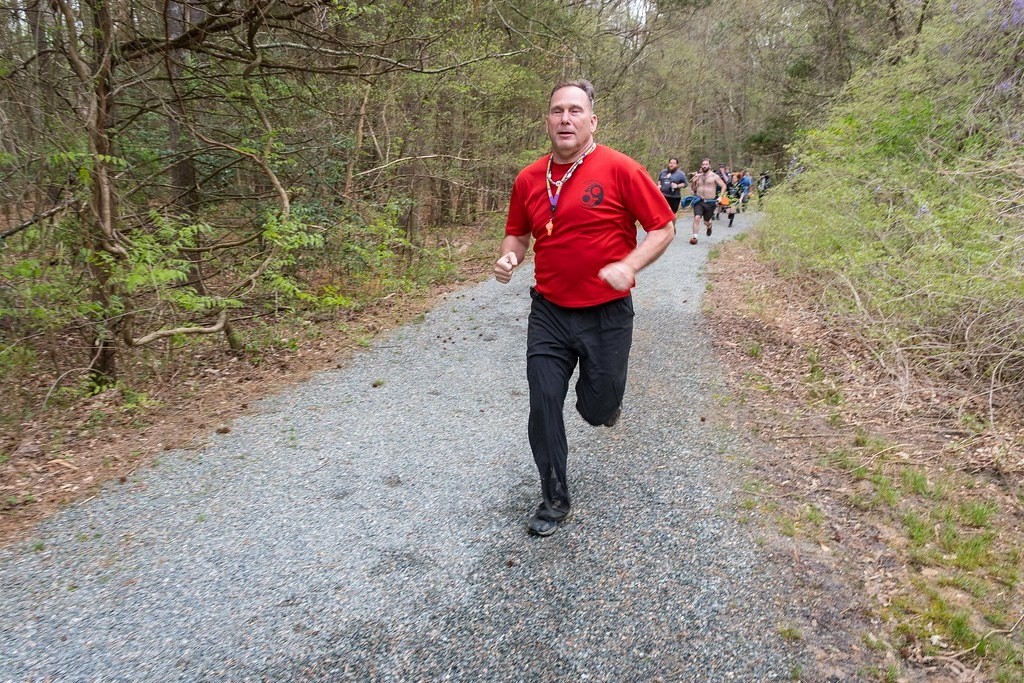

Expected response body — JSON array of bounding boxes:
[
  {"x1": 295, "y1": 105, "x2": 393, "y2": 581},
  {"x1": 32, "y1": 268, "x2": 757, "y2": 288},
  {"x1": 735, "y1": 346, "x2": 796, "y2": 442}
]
[
  {"x1": 712, "y1": 212, "x2": 719, "y2": 218},
  {"x1": 728, "y1": 222, "x2": 732, "y2": 226}
]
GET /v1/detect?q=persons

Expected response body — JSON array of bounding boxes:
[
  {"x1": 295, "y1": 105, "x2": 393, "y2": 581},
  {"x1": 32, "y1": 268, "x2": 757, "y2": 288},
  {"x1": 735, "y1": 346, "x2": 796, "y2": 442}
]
[
  {"x1": 492, "y1": 80, "x2": 675, "y2": 536},
  {"x1": 657, "y1": 157, "x2": 771, "y2": 245}
]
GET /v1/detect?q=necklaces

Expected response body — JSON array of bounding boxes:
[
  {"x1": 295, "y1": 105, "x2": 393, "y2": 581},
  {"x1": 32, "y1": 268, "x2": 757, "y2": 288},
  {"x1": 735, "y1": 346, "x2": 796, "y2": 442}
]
[{"x1": 547, "y1": 142, "x2": 596, "y2": 185}]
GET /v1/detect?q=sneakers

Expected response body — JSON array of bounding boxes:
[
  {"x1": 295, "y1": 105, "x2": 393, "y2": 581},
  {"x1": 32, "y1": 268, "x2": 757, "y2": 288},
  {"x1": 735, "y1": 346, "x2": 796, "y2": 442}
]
[
  {"x1": 604, "y1": 402, "x2": 622, "y2": 427},
  {"x1": 527, "y1": 514, "x2": 562, "y2": 536},
  {"x1": 690, "y1": 237, "x2": 697, "y2": 244},
  {"x1": 707, "y1": 225, "x2": 712, "y2": 236}
]
